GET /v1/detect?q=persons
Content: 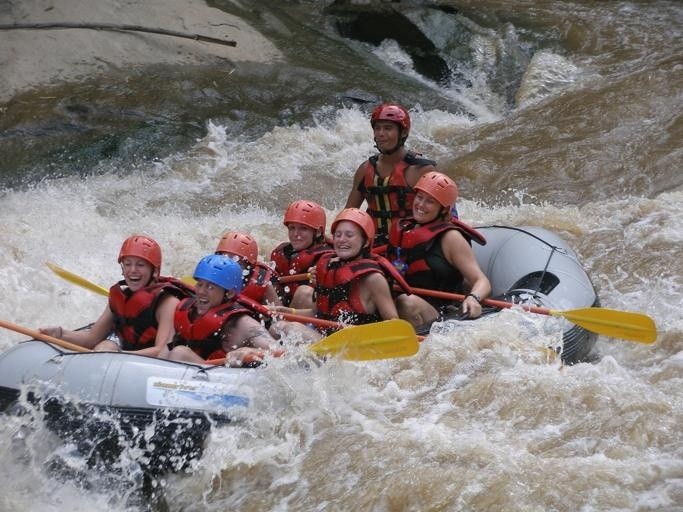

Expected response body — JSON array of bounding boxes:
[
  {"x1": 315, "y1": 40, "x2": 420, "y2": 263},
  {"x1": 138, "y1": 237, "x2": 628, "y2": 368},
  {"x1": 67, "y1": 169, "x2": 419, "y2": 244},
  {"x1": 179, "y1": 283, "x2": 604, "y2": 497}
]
[
  {"x1": 387, "y1": 172, "x2": 492, "y2": 329},
  {"x1": 259, "y1": 206, "x2": 399, "y2": 343},
  {"x1": 269, "y1": 200, "x2": 335, "y2": 306},
  {"x1": 340, "y1": 103, "x2": 440, "y2": 254},
  {"x1": 38, "y1": 235, "x2": 182, "y2": 359},
  {"x1": 214, "y1": 230, "x2": 286, "y2": 329},
  {"x1": 168, "y1": 254, "x2": 285, "y2": 369}
]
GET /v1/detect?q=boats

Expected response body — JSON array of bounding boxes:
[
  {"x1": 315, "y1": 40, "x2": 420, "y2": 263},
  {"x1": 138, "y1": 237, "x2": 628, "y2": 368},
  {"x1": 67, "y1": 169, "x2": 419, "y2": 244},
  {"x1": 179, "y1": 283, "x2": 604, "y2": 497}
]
[{"x1": 0, "y1": 225, "x2": 599, "y2": 470}]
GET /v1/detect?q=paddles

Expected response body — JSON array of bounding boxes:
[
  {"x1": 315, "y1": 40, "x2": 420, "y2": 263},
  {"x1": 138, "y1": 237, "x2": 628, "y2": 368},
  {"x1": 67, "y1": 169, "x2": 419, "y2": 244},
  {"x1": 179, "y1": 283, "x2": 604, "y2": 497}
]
[
  {"x1": 47, "y1": 263, "x2": 109, "y2": 296},
  {"x1": 179, "y1": 271, "x2": 313, "y2": 288},
  {"x1": 201, "y1": 318, "x2": 418, "y2": 364},
  {"x1": 394, "y1": 284, "x2": 657, "y2": 345}
]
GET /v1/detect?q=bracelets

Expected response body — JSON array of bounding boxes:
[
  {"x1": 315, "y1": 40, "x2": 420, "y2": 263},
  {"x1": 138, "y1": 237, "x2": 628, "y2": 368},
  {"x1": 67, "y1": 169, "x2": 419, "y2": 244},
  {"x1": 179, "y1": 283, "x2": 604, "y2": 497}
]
[
  {"x1": 465, "y1": 293, "x2": 481, "y2": 303},
  {"x1": 58, "y1": 326, "x2": 62, "y2": 339}
]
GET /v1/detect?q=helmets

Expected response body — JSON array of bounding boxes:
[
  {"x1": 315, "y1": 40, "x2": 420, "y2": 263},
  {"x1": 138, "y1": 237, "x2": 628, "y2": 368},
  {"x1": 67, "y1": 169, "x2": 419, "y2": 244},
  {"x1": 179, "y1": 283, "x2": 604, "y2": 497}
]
[
  {"x1": 215, "y1": 231, "x2": 258, "y2": 269},
  {"x1": 371, "y1": 103, "x2": 410, "y2": 137},
  {"x1": 284, "y1": 200, "x2": 326, "y2": 237},
  {"x1": 193, "y1": 255, "x2": 243, "y2": 294},
  {"x1": 414, "y1": 171, "x2": 458, "y2": 214},
  {"x1": 331, "y1": 207, "x2": 375, "y2": 248},
  {"x1": 118, "y1": 234, "x2": 161, "y2": 276}
]
